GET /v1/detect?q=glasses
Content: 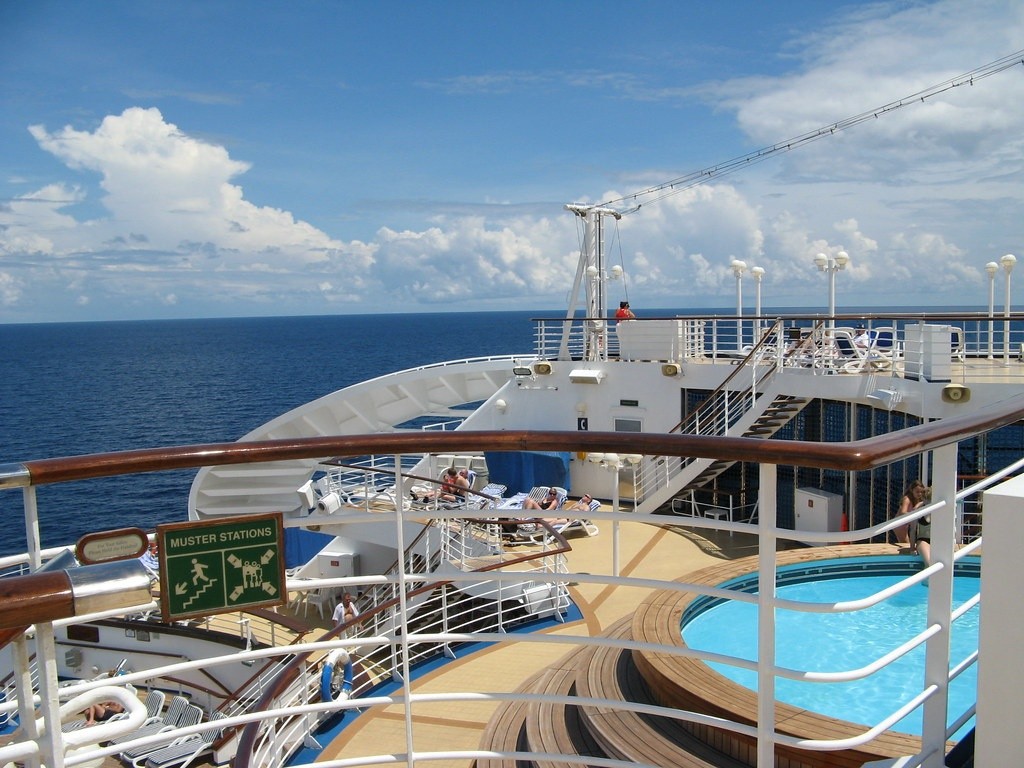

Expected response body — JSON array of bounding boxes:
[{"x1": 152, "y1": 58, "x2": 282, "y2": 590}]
[
  {"x1": 550, "y1": 493, "x2": 556, "y2": 496},
  {"x1": 585, "y1": 495, "x2": 591, "y2": 499}
]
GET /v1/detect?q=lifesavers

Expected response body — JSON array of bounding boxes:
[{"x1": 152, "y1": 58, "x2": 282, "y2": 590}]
[{"x1": 321, "y1": 648, "x2": 354, "y2": 713}]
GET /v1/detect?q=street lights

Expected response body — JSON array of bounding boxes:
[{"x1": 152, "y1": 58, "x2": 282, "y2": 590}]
[
  {"x1": 812, "y1": 251, "x2": 850, "y2": 374},
  {"x1": 1000, "y1": 253, "x2": 1017, "y2": 366},
  {"x1": 729, "y1": 259, "x2": 747, "y2": 354},
  {"x1": 751, "y1": 266, "x2": 766, "y2": 353},
  {"x1": 587, "y1": 265, "x2": 623, "y2": 363},
  {"x1": 986, "y1": 262, "x2": 999, "y2": 360}
]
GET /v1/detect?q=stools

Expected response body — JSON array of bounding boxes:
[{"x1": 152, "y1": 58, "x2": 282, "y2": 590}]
[{"x1": 703, "y1": 509, "x2": 729, "y2": 532}]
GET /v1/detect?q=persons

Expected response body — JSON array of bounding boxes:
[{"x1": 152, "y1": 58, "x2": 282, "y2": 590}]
[
  {"x1": 615, "y1": 302, "x2": 635, "y2": 323},
  {"x1": 85, "y1": 669, "x2": 123, "y2": 726},
  {"x1": 410, "y1": 468, "x2": 470, "y2": 503},
  {"x1": 797, "y1": 322, "x2": 871, "y2": 354},
  {"x1": 533, "y1": 494, "x2": 592, "y2": 528},
  {"x1": 518, "y1": 487, "x2": 558, "y2": 520},
  {"x1": 332, "y1": 592, "x2": 363, "y2": 640},
  {"x1": 889, "y1": 479, "x2": 932, "y2": 568}
]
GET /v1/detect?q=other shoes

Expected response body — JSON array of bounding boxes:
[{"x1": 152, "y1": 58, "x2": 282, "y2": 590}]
[
  {"x1": 423, "y1": 496, "x2": 430, "y2": 503},
  {"x1": 410, "y1": 490, "x2": 418, "y2": 500}
]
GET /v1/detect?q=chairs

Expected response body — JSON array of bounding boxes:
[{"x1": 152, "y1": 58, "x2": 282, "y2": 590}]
[
  {"x1": 62, "y1": 686, "x2": 230, "y2": 768},
  {"x1": 354, "y1": 471, "x2": 602, "y2": 546},
  {"x1": 743, "y1": 326, "x2": 964, "y2": 374},
  {"x1": 288, "y1": 573, "x2": 349, "y2": 619}
]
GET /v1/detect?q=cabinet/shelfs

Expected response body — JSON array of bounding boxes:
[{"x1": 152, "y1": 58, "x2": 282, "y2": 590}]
[{"x1": 794, "y1": 487, "x2": 844, "y2": 547}]
